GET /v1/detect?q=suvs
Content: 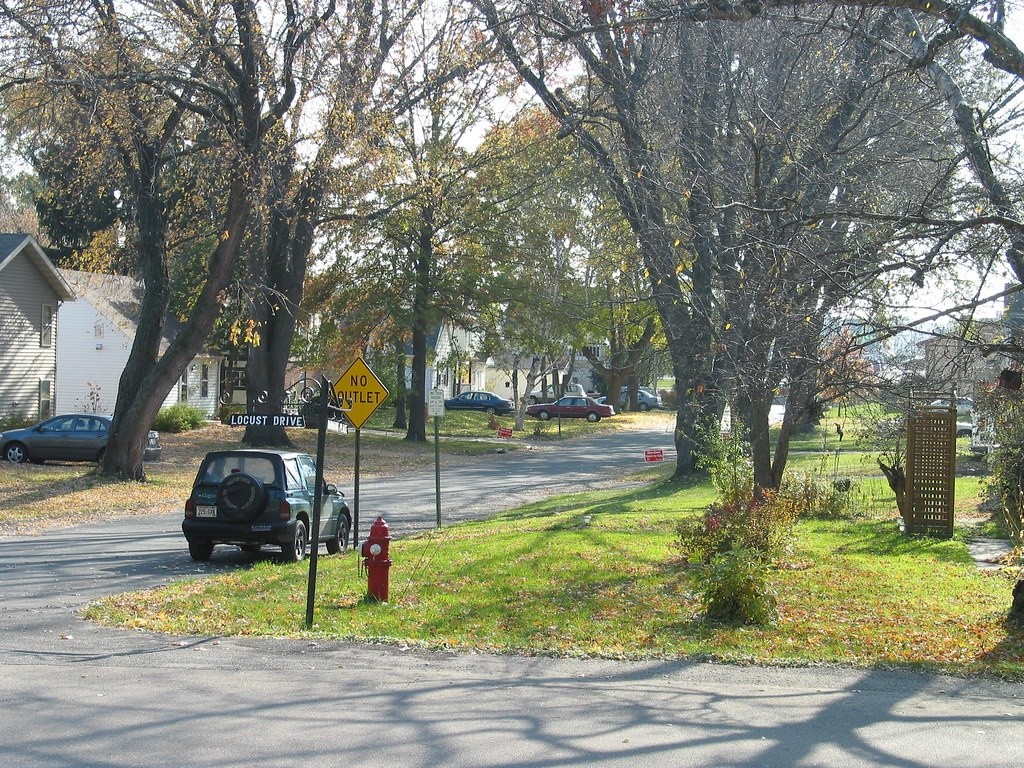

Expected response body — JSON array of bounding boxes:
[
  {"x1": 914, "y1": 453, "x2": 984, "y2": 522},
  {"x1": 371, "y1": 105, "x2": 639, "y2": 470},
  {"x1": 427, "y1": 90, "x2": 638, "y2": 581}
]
[{"x1": 181, "y1": 449, "x2": 352, "y2": 562}]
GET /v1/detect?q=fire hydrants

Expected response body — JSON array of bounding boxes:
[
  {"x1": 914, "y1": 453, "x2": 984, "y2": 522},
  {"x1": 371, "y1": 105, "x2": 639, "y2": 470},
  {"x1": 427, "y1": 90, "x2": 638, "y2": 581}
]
[{"x1": 357, "y1": 514, "x2": 393, "y2": 604}]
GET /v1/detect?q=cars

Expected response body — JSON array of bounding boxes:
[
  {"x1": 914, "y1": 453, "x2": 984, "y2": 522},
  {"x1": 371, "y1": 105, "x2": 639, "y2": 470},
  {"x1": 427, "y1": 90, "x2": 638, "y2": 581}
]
[
  {"x1": 593, "y1": 389, "x2": 664, "y2": 412},
  {"x1": 443, "y1": 391, "x2": 515, "y2": 417},
  {"x1": 928, "y1": 396, "x2": 976, "y2": 414},
  {"x1": 0, "y1": 414, "x2": 163, "y2": 465},
  {"x1": 525, "y1": 395, "x2": 617, "y2": 423}
]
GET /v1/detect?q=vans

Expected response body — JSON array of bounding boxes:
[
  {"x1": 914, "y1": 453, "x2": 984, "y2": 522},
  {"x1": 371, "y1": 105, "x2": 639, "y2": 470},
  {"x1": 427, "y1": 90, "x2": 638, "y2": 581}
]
[{"x1": 520, "y1": 384, "x2": 587, "y2": 405}]
